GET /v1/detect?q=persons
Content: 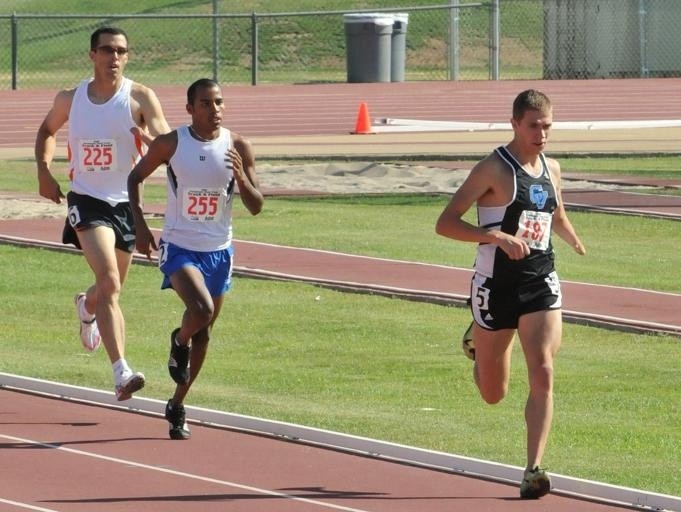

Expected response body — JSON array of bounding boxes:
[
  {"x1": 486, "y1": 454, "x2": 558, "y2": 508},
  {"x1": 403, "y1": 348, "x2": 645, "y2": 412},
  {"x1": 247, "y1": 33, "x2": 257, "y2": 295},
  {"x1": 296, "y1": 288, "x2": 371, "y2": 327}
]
[
  {"x1": 35, "y1": 27, "x2": 175, "y2": 402},
  {"x1": 434, "y1": 90, "x2": 588, "y2": 497},
  {"x1": 127, "y1": 77, "x2": 263, "y2": 439}
]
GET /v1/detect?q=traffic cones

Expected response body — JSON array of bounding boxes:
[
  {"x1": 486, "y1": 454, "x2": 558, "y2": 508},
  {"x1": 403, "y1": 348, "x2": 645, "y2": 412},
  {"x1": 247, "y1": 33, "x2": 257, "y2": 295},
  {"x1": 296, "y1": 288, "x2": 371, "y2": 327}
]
[{"x1": 350, "y1": 101, "x2": 375, "y2": 134}]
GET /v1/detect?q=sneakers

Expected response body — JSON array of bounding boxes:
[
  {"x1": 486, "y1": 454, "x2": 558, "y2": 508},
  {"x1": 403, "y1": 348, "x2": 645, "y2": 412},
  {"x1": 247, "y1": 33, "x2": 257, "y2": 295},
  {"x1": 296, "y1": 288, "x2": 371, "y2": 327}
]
[
  {"x1": 520, "y1": 466, "x2": 551, "y2": 499},
  {"x1": 165, "y1": 398, "x2": 192, "y2": 439},
  {"x1": 74, "y1": 292, "x2": 101, "y2": 353},
  {"x1": 168, "y1": 327, "x2": 193, "y2": 386},
  {"x1": 115, "y1": 371, "x2": 146, "y2": 401},
  {"x1": 462, "y1": 319, "x2": 475, "y2": 361}
]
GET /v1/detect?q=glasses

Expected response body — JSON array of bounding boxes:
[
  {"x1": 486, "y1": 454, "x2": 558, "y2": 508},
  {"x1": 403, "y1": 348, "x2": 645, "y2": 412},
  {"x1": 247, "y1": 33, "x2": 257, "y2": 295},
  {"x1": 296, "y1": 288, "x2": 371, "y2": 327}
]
[{"x1": 91, "y1": 45, "x2": 130, "y2": 57}]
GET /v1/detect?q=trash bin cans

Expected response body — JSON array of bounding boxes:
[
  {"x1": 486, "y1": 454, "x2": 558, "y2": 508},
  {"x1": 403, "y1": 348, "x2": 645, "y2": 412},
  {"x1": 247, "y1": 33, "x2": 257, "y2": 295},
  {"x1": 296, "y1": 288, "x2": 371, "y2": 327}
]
[{"x1": 343, "y1": 13, "x2": 409, "y2": 83}]
